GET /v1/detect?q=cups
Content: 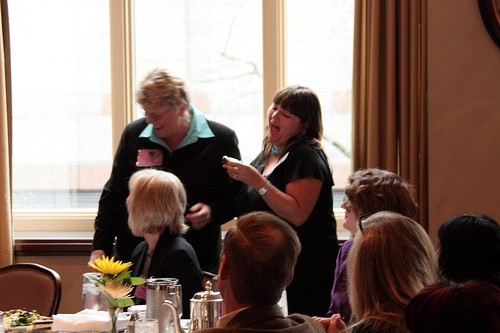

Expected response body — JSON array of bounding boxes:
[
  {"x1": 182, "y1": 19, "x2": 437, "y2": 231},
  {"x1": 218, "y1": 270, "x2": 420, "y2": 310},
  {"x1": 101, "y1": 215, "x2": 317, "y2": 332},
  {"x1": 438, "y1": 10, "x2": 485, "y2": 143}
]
[{"x1": 81, "y1": 272, "x2": 106, "y2": 311}]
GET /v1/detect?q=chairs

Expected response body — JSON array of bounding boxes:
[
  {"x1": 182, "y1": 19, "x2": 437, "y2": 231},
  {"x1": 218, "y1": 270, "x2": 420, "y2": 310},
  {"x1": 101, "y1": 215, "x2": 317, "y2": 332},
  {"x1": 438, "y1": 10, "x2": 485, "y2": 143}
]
[{"x1": 0, "y1": 263, "x2": 63, "y2": 316}]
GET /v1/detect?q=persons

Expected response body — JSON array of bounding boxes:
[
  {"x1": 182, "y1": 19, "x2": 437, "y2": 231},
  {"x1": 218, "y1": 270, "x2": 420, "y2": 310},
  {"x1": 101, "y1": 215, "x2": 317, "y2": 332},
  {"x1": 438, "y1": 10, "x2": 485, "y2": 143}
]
[
  {"x1": 188, "y1": 211, "x2": 325, "y2": 333},
  {"x1": 312, "y1": 168, "x2": 500, "y2": 333},
  {"x1": 222, "y1": 84, "x2": 340, "y2": 325},
  {"x1": 88, "y1": 69, "x2": 248, "y2": 276},
  {"x1": 116, "y1": 168, "x2": 205, "y2": 319}
]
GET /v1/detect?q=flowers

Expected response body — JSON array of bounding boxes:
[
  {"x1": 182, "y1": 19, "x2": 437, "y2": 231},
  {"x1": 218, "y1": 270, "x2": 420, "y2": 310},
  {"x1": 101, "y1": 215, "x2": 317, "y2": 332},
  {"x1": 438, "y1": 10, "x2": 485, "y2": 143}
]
[{"x1": 82, "y1": 254, "x2": 147, "y2": 333}]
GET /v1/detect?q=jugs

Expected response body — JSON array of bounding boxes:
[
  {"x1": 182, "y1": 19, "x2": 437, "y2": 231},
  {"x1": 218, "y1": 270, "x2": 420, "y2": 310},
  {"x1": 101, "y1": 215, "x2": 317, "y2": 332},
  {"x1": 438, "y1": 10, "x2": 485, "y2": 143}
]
[{"x1": 147, "y1": 278, "x2": 185, "y2": 333}]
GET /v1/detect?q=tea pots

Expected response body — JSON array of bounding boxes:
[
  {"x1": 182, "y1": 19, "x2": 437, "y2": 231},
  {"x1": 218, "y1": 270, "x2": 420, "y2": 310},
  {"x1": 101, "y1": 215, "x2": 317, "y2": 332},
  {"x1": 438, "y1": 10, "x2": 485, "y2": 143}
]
[{"x1": 161, "y1": 280, "x2": 225, "y2": 333}]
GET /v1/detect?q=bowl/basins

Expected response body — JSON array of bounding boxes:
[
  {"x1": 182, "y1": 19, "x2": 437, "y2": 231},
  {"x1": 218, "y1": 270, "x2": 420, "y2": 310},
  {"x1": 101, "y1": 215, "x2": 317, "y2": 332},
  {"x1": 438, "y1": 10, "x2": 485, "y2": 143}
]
[{"x1": 127, "y1": 305, "x2": 147, "y2": 318}]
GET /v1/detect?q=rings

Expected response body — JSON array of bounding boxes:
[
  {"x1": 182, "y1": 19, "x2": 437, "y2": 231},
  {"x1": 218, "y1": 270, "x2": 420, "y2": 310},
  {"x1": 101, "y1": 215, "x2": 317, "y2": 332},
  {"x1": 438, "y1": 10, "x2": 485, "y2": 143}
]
[{"x1": 234, "y1": 174, "x2": 239, "y2": 178}]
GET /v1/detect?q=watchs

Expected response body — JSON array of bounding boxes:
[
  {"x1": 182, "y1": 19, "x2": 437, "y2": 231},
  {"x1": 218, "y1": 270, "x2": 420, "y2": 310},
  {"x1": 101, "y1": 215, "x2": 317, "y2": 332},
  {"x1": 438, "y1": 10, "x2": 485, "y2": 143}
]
[{"x1": 258, "y1": 181, "x2": 273, "y2": 196}]
